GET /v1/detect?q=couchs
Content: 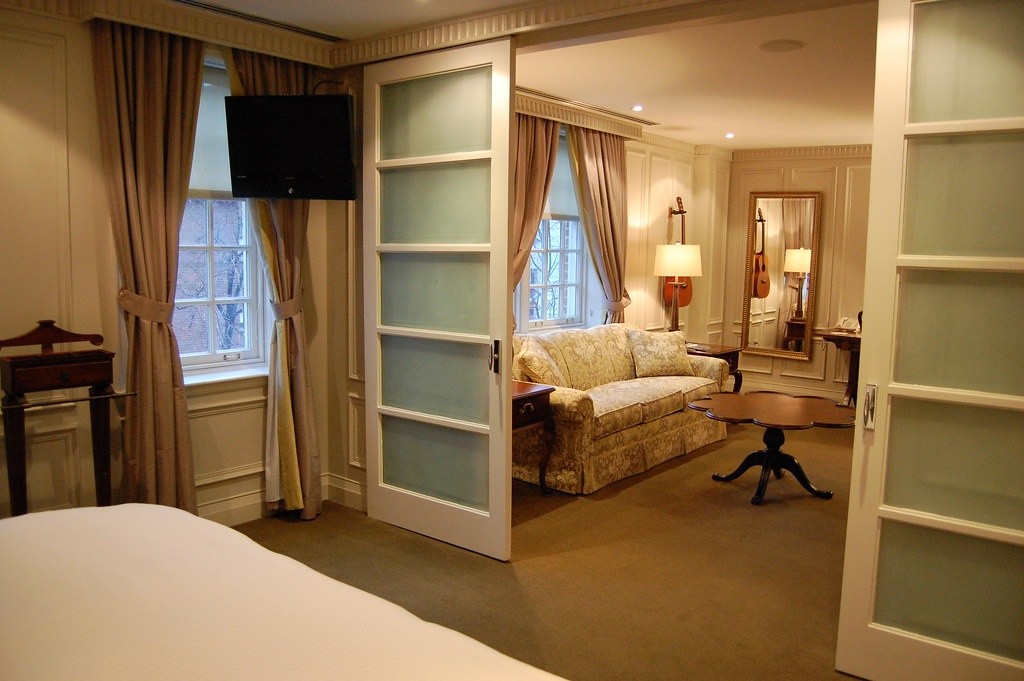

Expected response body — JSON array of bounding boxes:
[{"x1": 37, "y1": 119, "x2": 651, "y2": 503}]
[{"x1": 512, "y1": 323, "x2": 729, "y2": 495}]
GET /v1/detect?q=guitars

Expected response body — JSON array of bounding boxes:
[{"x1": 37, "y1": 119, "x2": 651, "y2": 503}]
[
  {"x1": 663, "y1": 197, "x2": 694, "y2": 308},
  {"x1": 753, "y1": 206, "x2": 770, "y2": 297}
]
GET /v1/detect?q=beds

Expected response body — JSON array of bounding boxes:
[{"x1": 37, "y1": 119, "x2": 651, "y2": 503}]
[{"x1": 0, "y1": 504, "x2": 569, "y2": 681}]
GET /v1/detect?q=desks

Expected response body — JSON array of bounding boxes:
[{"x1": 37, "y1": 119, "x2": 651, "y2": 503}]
[
  {"x1": 511, "y1": 381, "x2": 556, "y2": 496},
  {"x1": 782, "y1": 322, "x2": 805, "y2": 352},
  {"x1": 685, "y1": 342, "x2": 742, "y2": 392},
  {"x1": 824, "y1": 332, "x2": 862, "y2": 405}
]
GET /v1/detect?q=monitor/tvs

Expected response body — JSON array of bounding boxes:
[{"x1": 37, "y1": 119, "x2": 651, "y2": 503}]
[{"x1": 225, "y1": 94, "x2": 358, "y2": 200}]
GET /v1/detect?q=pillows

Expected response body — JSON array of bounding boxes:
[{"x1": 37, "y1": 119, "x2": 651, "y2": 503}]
[
  {"x1": 628, "y1": 328, "x2": 694, "y2": 377},
  {"x1": 518, "y1": 336, "x2": 568, "y2": 388}
]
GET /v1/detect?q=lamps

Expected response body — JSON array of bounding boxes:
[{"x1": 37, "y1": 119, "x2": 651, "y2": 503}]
[
  {"x1": 784, "y1": 247, "x2": 811, "y2": 321},
  {"x1": 654, "y1": 241, "x2": 701, "y2": 332}
]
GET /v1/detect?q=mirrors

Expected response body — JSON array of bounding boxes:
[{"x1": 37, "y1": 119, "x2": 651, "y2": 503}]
[{"x1": 747, "y1": 197, "x2": 816, "y2": 353}]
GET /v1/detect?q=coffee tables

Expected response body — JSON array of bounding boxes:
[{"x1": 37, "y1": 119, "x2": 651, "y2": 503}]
[{"x1": 687, "y1": 391, "x2": 857, "y2": 505}]
